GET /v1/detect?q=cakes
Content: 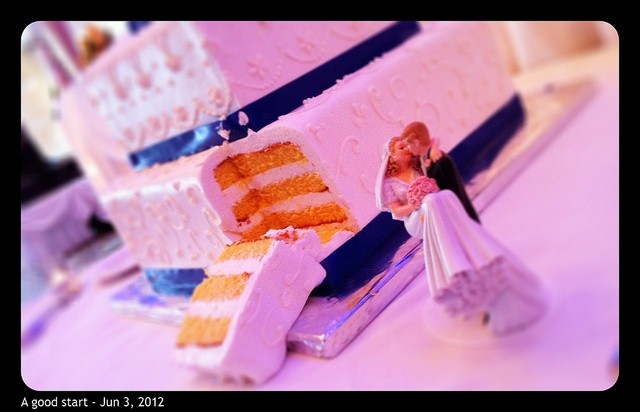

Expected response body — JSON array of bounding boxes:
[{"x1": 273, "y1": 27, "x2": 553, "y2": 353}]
[
  {"x1": 172, "y1": 237, "x2": 326, "y2": 386},
  {"x1": 54, "y1": 21, "x2": 525, "y2": 298}
]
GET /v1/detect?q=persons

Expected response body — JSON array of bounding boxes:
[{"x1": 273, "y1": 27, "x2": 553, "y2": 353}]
[
  {"x1": 400, "y1": 121, "x2": 480, "y2": 223},
  {"x1": 374, "y1": 136, "x2": 547, "y2": 337}
]
